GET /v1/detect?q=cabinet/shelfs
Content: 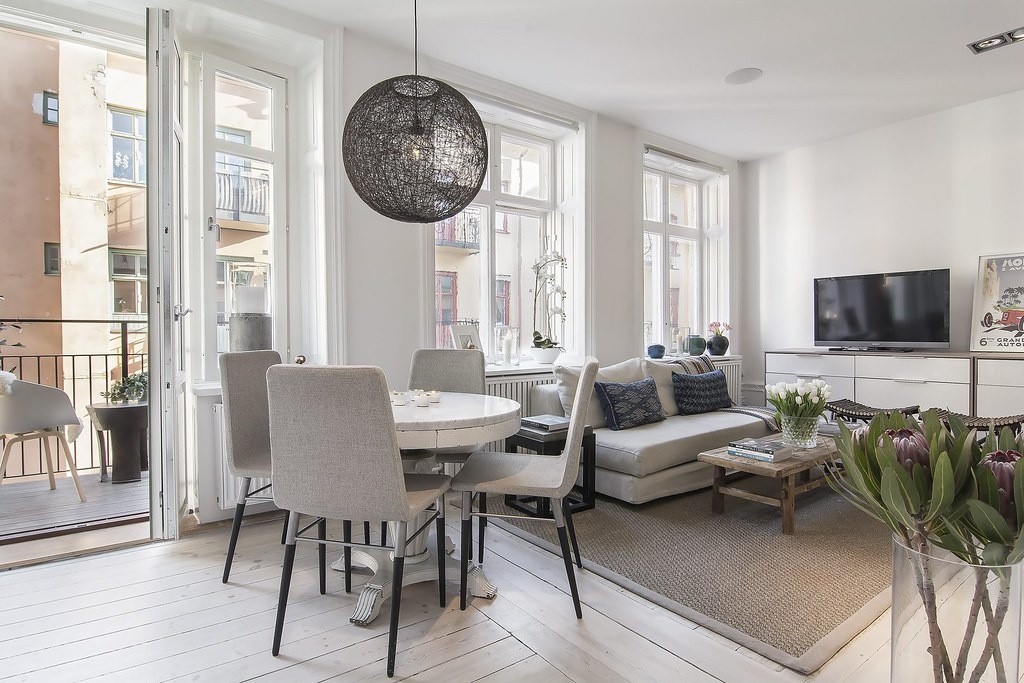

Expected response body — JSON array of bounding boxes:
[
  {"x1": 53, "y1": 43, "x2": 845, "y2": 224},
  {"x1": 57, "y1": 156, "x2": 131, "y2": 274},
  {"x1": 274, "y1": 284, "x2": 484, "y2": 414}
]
[{"x1": 764, "y1": 349, "x2": 1024, "y2": 449}]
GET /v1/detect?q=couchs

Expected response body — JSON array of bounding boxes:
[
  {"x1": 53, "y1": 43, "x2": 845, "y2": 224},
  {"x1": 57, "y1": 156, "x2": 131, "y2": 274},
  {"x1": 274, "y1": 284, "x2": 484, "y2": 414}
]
[{"x1": 531, "y1": 357, "x2": 781, "y2": 504}]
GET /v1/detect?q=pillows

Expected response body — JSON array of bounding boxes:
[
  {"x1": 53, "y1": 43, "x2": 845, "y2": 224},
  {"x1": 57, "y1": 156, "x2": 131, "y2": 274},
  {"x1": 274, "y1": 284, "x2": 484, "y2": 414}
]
[
  {"x1": 594, "y1": 376, "x2": 667, "y2": 431},
  {"x1": 672, "y1": 368, "x2": 731, "y2": 416}
]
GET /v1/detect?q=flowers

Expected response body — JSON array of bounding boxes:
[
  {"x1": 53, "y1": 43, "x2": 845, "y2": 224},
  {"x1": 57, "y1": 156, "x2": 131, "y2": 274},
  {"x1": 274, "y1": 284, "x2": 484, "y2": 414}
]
[
  {"x1": 529, "y1": 250, "x2": 568, "y2": 352},
  {"x1": 99, "y1": 372, "x2": 148, "y2": 404},
  {"x1": 810, "y1": 408, "x2": 1024, "y2": 683},
  {"x1": 708, "y1": 322, "x2": 733, "y2": 335},
  {"x1": 765, "y1": 378, "x2": 833, "y2": 443}
]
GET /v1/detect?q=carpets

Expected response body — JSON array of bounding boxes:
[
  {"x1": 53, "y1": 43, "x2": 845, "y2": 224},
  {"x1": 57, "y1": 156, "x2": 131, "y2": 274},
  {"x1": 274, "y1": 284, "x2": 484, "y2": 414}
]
[{"x1": 450, "y1": 475, "x2": 980, "y2": 674}]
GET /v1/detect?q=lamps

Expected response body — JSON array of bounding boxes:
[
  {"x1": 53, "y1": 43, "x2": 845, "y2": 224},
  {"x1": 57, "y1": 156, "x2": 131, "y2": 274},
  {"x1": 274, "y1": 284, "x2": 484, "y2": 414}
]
[{"x1": 342, "y1": 1, "x2": 488, "y2": 223}]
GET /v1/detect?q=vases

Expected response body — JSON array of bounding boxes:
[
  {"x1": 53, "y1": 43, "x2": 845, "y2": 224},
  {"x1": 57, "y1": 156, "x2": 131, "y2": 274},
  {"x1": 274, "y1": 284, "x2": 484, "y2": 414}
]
[
  {"x1": 530, "y1": 348, "x2": 560, "y2": 363},
  {"x1": 781, "y1": 416, "x2": 820, "y2": 448},
  {"x1": 683, "y1": 334, "x2": 729, "y2": 355},
  {"x1": 890, "y1": 532, "x2": 1024, "y2": 683}
]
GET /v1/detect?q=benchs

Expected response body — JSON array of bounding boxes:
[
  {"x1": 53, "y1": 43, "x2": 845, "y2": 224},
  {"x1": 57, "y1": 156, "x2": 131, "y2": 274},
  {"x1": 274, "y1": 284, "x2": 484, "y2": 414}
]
[
  {"x1": 918, "y1": 408, "x2": 1023, "y2": 450},
  {"x1": 826, "y1": 398, "x2": 920, "y2": 424}
]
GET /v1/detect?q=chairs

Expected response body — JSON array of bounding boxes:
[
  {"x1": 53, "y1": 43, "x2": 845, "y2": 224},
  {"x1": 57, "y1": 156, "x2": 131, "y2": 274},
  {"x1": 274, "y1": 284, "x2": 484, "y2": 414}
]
[
  {"x1": 0, "y1": 379, "x2": 86, "y2": 502},
  {"x1": 219, "y1": 348, "x2": 598, "y2": 678}
]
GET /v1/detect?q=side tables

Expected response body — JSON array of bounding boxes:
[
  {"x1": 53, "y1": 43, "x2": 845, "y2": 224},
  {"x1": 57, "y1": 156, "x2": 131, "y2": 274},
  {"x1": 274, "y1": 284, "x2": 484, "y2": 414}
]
[{"x1": 505, "y1": 433, "x2": 596, "y2": 518}]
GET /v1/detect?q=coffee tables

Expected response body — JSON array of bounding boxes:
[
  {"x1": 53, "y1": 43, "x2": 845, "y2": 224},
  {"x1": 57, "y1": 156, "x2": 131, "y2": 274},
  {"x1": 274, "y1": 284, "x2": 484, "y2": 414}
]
[{"x1": 697, "y1": 432, "x2": 845, "y2": 535}]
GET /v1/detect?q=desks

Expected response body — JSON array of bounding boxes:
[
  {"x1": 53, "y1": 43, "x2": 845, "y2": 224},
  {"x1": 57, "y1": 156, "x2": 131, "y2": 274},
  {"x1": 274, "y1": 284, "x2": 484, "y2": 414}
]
[
  {"x1": 85, "y1": 402, "x2": 149, "y2": 484},
  {"x1": 330, "y1": 392, "x2": 521, "y2": 625}
]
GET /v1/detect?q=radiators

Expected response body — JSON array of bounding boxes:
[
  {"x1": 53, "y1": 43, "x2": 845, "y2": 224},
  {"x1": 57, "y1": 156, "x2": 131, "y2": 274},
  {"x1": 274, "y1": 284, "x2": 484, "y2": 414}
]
[
  {"x1": 212, "y1": 403, "x2": 273, "y2": 510},
  {"x1": 445, "y1": 360, "x2": 743, "y2": 477}
]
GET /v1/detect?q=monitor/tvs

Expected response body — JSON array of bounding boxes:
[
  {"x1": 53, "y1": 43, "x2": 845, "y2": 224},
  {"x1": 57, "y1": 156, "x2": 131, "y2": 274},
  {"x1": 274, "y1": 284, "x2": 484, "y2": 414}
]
[{"x1": 814, "y1": 268, "x2": 950, "y2": 349}]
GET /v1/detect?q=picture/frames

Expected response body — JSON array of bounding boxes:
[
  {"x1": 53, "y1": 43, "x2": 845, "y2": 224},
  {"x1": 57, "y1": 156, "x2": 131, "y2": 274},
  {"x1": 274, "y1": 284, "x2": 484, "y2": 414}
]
[{"x1": 449, "y1": 325, "x2": 483, "y2": 352}]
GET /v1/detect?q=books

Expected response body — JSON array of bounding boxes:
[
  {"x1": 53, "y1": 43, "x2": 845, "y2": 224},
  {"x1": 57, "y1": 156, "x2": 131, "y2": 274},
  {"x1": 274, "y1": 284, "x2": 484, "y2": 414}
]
[{"x1": 728, "y1": 438, "x2": 792, "y2": 462}]
